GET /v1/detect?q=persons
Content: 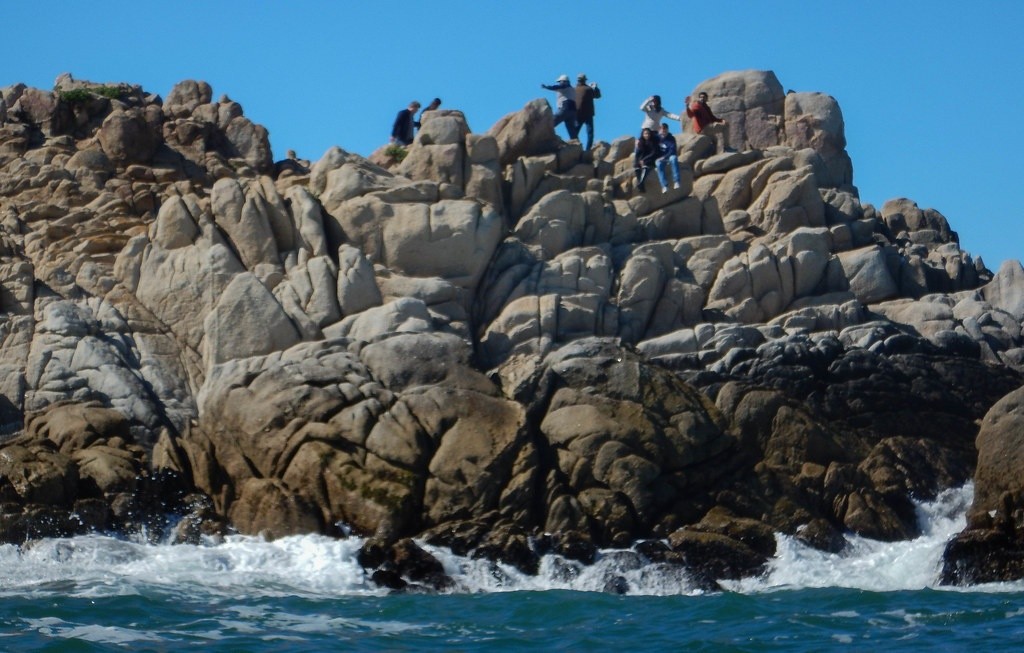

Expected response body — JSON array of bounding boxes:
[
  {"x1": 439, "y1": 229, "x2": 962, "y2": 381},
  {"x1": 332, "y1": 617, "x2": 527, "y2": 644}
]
[
  {"x1": 685, "y1": 92, "x2": 729, "y2": 135},
  {"x1": 390, "y1": 98, "x2": 441, "y2": 147},
  {"x1": 541, "y1": 75, "x2": 578, "y2": 140},
  {"x1": 575, "y1": 74, "x2": 601, "y2": 151},
  {"x1": 638, "y1": 95, "x2": 681, "y2": 140},
  {"x1": 633, "y1": 123, "x2": 680, "y2": 193}
]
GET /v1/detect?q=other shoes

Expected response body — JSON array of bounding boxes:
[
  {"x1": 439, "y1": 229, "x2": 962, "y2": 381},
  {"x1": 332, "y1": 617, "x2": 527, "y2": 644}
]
[
  {"x1": 662, "y1": 184, "x2": 670, "y2": 194},
  {"x1": 674, "y1": 182, "x2": 680, "y2": 190},
  {"x1": 723, "y1": 146, "x2": 737, "y2": 153},
  {"x1": 637, "y1": 183, "x2": 645, "y2": 193}
]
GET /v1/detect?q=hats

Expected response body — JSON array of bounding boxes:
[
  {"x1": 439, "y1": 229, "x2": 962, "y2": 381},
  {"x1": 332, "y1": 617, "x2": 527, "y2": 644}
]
[
  {"x1": 556, "y1": 75, "x2": 569, "y2": 82},
  {"x1": 577, "y1": 74, "x2": 588, "y2": 82}
]
